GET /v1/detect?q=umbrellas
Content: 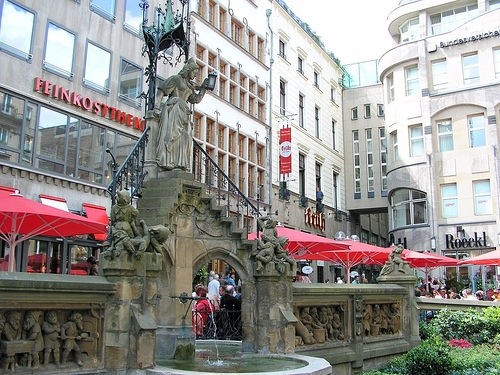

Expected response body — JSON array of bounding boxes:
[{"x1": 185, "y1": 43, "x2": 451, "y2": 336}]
[
  {"x1": 299, "y1": 233, "x2": 406, "y2": 284},
  {"x1": 243, "y1": 222, "x2": 352, "y2": 286},
  {"x1": 455, "y1": 244, "x2": 500, "y2": 269},
  {"x1": 2, "y1": 188, "x2": 109, "y2": 272},
  {"x1": 409, "y1": 249, "x2": 460, "y2": 296},
  {"x1": 383, "y1": 242, "x2": 444, "y2": 287}
]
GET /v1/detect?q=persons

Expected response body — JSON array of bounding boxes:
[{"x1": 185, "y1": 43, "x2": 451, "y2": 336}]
[
  {"x1": 154, "y1": 56, "x2": 206, "y2": 173},
  {"x1": 381, "y1": 239, "x2": 406, "y2": 276},
  {"x1": 300, "y1": 302, "x2": 401, "y2": 344},
  {"x1": 104, "y1": 189, "x2": 172, "y2": 261},
  {"x1": 416, "y1": 274, "x2": 500, "y2": 304},
  {"x1": 293, "y1": 267, "x2": 362, "y2": 285},
  {"x1": 192, "y1": 263, "x2": 243, "y2": 340},
  {"x1": 254, "y1": 214, "x2": 295, "y2": 274},
  {"x1": 1, "y1": 309, "x2": 88, "y2": 374}
]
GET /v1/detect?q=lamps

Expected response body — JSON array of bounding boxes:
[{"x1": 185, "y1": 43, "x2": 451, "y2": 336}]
[{"x1": 430, "y1": 237, "x2": 435, "y2": 251}]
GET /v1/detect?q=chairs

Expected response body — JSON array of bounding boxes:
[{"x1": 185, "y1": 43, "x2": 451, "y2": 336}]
[{"x1": 0, "y1": 249, "x2": 96, "y2": 280}]
